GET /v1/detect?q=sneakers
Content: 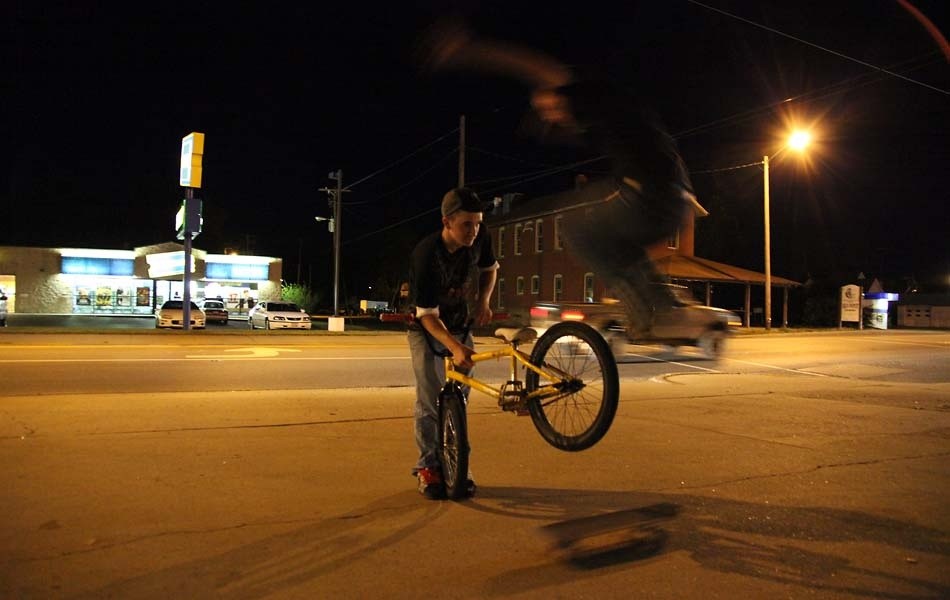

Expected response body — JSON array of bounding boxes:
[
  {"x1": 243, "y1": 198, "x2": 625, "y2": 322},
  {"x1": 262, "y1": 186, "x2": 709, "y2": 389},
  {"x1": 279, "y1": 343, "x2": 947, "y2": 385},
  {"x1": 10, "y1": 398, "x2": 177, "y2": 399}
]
[
  {"x1": 416, "y1": 467, "x2": 445, "y2": 501},
  {"x1": 450, "y1": 470, "x2": 477, "y2": 496}
]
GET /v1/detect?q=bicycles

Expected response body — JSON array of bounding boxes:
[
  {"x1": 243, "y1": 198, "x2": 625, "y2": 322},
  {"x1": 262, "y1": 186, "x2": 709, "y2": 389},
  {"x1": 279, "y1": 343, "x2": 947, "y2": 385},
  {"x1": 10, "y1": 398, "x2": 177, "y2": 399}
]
[{"x1": 379, "y1": 299, "x2": 620, "y2": 501}]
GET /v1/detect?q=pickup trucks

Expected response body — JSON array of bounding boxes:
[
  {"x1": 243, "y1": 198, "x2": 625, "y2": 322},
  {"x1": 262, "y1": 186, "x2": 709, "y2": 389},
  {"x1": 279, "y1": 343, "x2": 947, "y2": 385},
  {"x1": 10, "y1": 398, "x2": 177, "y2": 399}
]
[{"x1": 527, "y1": 273, "x2": 742, "y2": 362}]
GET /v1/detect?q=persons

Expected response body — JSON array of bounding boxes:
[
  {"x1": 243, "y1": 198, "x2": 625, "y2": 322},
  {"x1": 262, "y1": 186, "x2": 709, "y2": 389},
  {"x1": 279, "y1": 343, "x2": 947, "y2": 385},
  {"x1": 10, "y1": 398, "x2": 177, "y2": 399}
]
[
  {"x1": 443, "y1": 34, "x2": 708, "y2": 342},
  {"x1": 408, "y1": 189, "x2": 500, "y2": 501}
]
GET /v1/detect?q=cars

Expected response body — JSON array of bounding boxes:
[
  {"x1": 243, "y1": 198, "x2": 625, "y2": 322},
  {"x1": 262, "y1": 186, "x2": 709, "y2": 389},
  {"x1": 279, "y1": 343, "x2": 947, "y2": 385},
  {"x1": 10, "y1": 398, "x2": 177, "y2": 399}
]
[
  {"x1": 153, "y1": 299, "x2": 207, "y2": 329},
  {"x1": 247, "y1": 300, "x2": 312, "y2": 330},
  {"x1": 198, "y1": 299, "x2": 229, "y2": 326}
]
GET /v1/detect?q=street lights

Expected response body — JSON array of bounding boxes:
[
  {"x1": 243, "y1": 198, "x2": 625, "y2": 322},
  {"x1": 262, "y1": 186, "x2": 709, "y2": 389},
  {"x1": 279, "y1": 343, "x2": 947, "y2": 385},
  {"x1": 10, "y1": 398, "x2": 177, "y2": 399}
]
[
  {"x1": 764, "y1": 129, "x2": 812, "y2": 332},
  {"x1": 315, "y1": 217, "x2": 341, "y2": 316}
]
